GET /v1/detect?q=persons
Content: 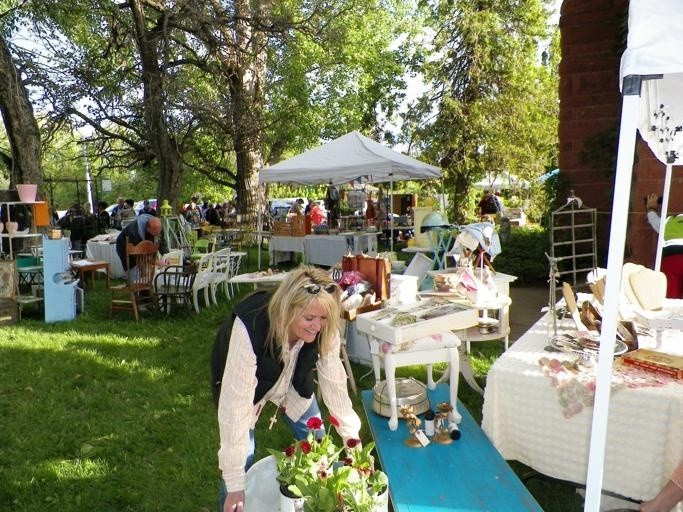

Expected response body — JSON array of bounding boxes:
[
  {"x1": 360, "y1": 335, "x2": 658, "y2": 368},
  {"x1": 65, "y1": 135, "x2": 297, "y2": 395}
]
[
  {"x1": 643, "y1": 192, "x2": 661, "y2": 236},
  {"x1": 208, "y1": 263, "x2": 362, "y2": 511},
  {"x1": 639, "y1": 455, "x2": 683, "y2": 512}
]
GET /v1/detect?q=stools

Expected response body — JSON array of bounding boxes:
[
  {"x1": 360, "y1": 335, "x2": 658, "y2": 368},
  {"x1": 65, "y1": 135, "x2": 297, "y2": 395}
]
[{"x1": 370, "y1": 331, "x2": 462, "y2": 431}]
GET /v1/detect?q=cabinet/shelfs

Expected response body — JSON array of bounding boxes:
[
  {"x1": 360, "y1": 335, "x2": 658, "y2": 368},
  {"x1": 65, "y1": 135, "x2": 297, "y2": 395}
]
[{"x1": 0, "y1": 201, "x2": 42, "y2": 321}]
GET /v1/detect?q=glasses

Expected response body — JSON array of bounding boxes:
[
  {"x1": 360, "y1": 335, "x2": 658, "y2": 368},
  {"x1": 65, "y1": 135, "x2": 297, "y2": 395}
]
[{"x1": 302, "y1": 283, "x2": 340, "y2": 295}]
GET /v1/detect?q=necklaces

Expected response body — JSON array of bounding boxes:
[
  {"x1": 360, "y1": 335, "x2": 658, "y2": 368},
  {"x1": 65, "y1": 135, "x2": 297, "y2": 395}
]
[{"x1": 268, "y1": 391, "x2": 284, "y2": 431}]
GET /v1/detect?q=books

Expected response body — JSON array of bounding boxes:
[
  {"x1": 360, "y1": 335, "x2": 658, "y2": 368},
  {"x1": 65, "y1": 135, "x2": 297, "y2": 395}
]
[{"x1": 621, "y1": 347, "x2": 682, "y2": 379}]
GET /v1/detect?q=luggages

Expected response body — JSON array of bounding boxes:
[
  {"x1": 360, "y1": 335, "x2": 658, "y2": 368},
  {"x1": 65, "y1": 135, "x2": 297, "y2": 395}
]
[{"x1": 341, "y1": 254, "x2": 391, "y2": 318}]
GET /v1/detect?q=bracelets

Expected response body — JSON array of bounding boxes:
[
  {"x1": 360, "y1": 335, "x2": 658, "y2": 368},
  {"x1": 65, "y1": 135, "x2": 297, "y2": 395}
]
[{"x1": 668, "y1": 476, "x2": 683, "y2": 491}]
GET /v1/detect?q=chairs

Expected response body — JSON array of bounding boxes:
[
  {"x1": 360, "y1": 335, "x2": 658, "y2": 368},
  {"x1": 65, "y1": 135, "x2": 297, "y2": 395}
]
[{"x1": 17, "y1": 236, "x2": 247, "y2": 320}]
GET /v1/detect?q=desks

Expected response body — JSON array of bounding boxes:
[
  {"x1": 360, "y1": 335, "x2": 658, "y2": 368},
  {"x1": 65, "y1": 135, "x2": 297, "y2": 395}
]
[
  {"x1": 481, "y1": 292, "x2": 683, "y2": 512},
  {"x1": 305, "y1": 232, "x2": 375, "y2": 267},
  {"x1": 427, "y1": 225, "x2": 496, "y2": 272},
  {"x1": 269, "y1": 235, "x2": 304, "y2": 267},
  {"x1": 426, "y1": 268, "x2": 519, "y2": 354}
]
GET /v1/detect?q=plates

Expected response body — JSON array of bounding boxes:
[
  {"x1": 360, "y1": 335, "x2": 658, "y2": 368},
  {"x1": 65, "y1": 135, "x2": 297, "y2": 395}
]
[{"x1": 549, "y1": 333, "x2": 628, "y2": 356}]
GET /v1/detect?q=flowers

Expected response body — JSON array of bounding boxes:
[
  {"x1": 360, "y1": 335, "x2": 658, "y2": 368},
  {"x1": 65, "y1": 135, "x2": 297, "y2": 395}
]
[{"x1": 265, "y1": 415, "x2": 388, "y2": 512}]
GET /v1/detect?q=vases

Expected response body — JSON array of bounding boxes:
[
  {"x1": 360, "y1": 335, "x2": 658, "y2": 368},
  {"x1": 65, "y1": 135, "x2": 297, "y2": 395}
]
[{"x1": 16, "y1": 183, "x2": 37, "y2": 203}]
